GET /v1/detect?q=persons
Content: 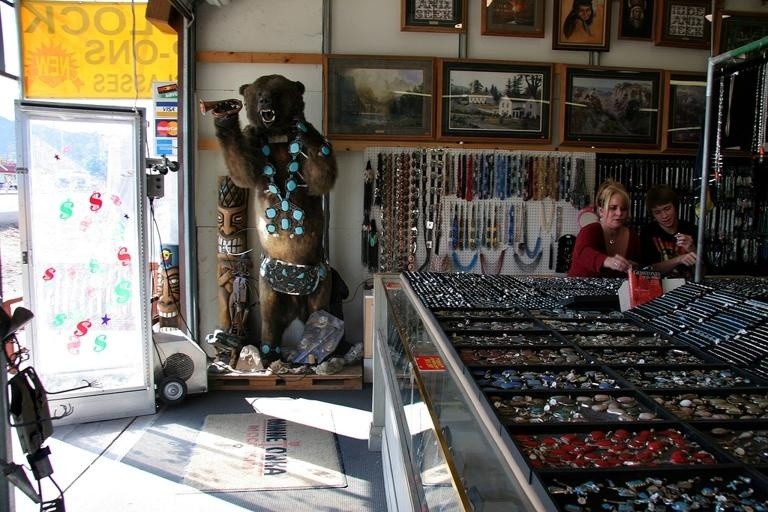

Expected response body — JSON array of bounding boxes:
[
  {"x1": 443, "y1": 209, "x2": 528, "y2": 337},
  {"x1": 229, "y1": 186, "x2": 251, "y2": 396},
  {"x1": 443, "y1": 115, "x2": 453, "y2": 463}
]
[
  {"x1": 567, "y1": 178, "x2": 641, "y2": 279},
  {"x1": 562, "y1": 2, "x2": 600, "y2": 43},
  {"x1": 640, "y1": 184, "x2": 706, "y2": 278}
]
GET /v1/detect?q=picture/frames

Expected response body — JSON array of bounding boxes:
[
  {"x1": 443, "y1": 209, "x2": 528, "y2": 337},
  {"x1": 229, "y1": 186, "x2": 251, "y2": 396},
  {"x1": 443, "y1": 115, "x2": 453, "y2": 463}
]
[
  {"x1": 320, "y1": 53, "x2": 437, "y2": 141},
  {"x1": 663, "y1": 69, "x2": 705, "y2": 152},
  {"x1": 618, "y1": 0, "x2": 656, "y2": 41},
  {"x1": 480, "y1": 0, "x2": 546, "y2": 38},
  {"x1": 713, "y1": 8, "x2": 766, "y2": 56},
  {"x1": 437, "y1": 56, "x2": 554, "y2": 144},
  {"x1": 552, "y1": 0, "x2": 613, "y2": 53},
  {"x1": 656, "y1": 0, "x2": 712, "y2": 51},
  {"x1": 398, "y1": 0, "x2": 468, "y2": 33},
  {"x1": 560, "y1": 64, "x2": 663, "y2": 149}
]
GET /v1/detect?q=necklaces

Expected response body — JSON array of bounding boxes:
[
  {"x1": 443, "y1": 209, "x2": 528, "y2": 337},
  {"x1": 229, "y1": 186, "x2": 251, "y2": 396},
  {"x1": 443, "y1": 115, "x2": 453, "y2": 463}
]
[
  {"x1": 255, "y1": 122, "x2": 307, "y2": 240},
  {"x1": 631, "y1": 281, "x2": 764, "y2": 376},
  {"x1": 607, "y1": 237, "x2": 617, "y2": 250},
  {"x1": 447, "y1": 200, "x2": 562, "y2": 276},
  {"x1": 360, "y1": 149, "x2": 444, "y2": 273},
  {"x1": 444, "y1": 152, "x2": 571, "y2": 202}
]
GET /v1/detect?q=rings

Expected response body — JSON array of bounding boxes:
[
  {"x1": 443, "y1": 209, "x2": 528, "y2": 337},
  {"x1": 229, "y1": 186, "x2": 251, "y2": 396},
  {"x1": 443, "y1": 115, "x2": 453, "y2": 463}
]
[
  {"x1": 677, "y1": 232, "x2": 681, "y2": 236},
  {"x1": 614, "y1": 262, "x2": 618, "y2": 266}
]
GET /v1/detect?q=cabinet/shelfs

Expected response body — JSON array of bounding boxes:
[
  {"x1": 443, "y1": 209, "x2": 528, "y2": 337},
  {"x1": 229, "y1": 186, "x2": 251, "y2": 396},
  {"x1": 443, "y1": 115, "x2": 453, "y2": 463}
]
[{"x1": 369, "y1": 271, "x2": 539, "y2": 512}]
